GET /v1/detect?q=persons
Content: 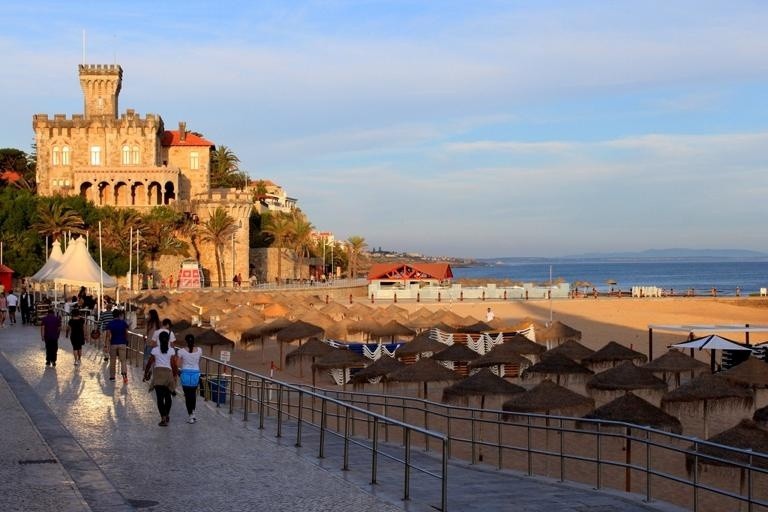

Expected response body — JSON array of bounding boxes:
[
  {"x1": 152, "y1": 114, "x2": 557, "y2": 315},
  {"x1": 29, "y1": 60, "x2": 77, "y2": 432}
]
[
  {"x1": 233, "y1": 273, "x2": 241, "y2": 286},
  {"x1": 486, "y1": 308, "x2": 495, "y2": 321},
  {"x1": 0, "y1": 273, "x2": 204, "y2": 427},
  {"x1": 310, "y1": 272, "x2": 334, "y2": 285}
]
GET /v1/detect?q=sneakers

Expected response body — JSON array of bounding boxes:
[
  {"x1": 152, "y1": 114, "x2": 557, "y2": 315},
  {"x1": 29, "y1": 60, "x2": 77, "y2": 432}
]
[
  {"x1": 158, "y1": 414, "x2": 197, "y2": 426},
  {"x1": 109, "y1": 371, "x2": 128, "y2": 382},
  {"x1": 46, "y1": 357, "x2": 81, "y2": 365}
]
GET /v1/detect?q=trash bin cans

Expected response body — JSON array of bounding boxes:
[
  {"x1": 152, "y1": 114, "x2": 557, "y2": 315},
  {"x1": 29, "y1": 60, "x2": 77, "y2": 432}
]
[{"x1": 199, "y1": 374, "x2": 228, "y2": 404}]
